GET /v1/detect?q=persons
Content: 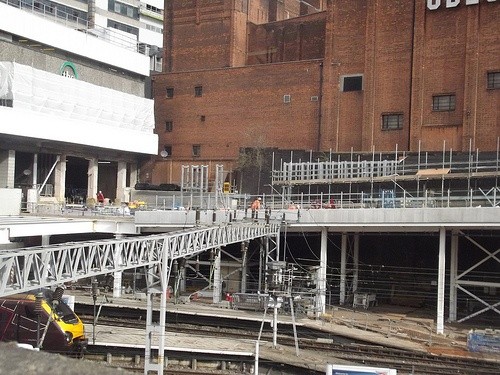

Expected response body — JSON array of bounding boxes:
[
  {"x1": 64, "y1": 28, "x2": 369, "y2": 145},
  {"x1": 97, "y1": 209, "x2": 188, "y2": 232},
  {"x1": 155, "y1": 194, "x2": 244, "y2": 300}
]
[
  {"x1": 251, "y1": 197, "x2": 261, "y2": 219},
  {"x1": 96, "y1": 191, "x2": 104, "y2": 204}
]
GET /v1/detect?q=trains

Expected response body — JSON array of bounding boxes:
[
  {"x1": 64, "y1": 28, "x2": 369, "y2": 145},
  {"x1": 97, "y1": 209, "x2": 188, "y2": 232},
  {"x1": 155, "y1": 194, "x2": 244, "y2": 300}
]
[{"x1": 0, "y1": 287, "x2": 88, "y2": 359}]
